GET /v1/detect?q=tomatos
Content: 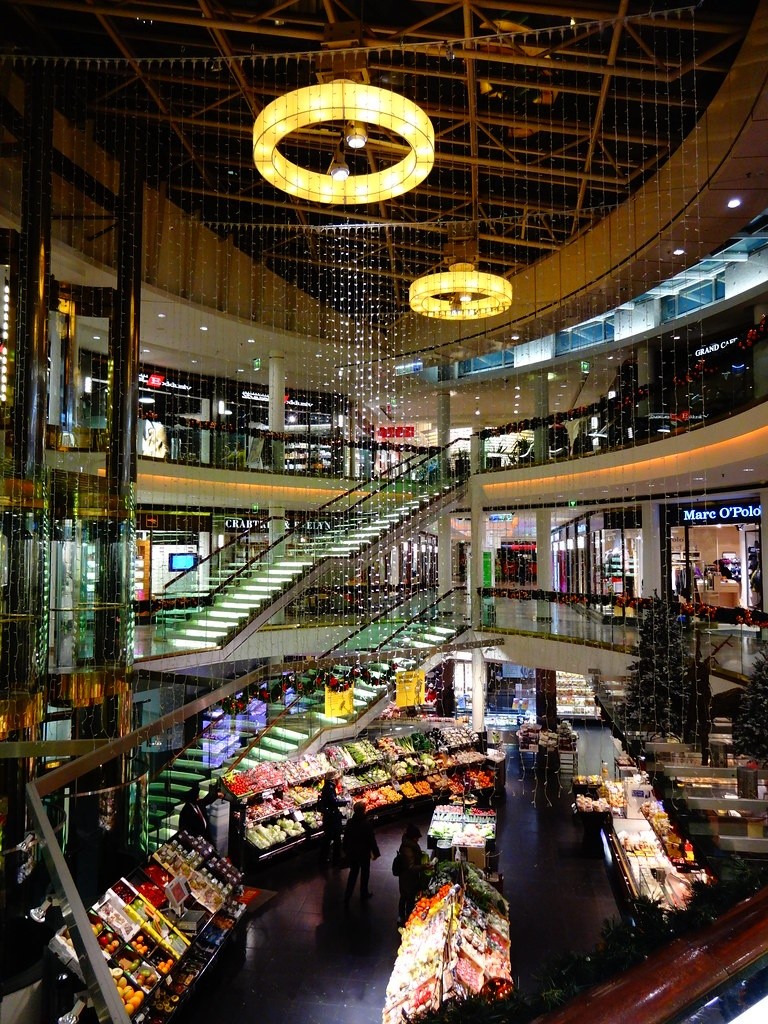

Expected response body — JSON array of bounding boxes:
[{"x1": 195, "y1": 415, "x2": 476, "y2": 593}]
[{"x1": 224, "y1": 776, "x2": 248, "y2": 794}]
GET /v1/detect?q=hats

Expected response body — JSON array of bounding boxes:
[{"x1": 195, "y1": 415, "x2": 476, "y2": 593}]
[{"x1": 407, "y1": 823, "x2": 422, "y2": 838}]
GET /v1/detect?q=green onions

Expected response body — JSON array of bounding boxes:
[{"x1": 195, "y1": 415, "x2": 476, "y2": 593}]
[{"x1": 397, "y1": 736, "x2": 415, "y2": 753}]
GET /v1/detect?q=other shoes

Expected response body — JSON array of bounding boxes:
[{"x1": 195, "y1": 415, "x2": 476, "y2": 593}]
[
  {"x1": 397, "y1": 917, "x2": 403, "y2": 924},
  {"x1": 360, "y1": 893, "x2": 372, "y2": 898}
]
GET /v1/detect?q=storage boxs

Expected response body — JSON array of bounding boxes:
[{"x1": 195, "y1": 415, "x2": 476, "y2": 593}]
[
  {"x1": 44, "y1": 828, "x2": 247, "y2": 1024},
  {"x1": 382, "y1": 863, "x2": 513, "y2": 1024},
  {"x1": 468, "y1": 848, "x2": 487, "y2": 869}
]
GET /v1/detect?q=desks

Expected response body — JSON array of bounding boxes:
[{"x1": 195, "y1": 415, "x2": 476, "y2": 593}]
[{"x1": 519, "y1": 748, "x2": 538, "y2": 770}]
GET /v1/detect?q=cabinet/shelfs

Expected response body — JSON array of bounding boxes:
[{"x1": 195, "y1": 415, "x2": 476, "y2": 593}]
[
  {"x1": 604, "y1": 801, "x2": 713, "y2": 927},
  {"x1": 218, "y1": 723, "x2": 496, "y2": 863},
  {"x1": 556, "y1": 672, "x2": 602, "y2": 720},
  {"x1": 610, "y1": 551, "x2": 740, "y2": 607},
  {"x1": 559, "y1": 750, "x2": 579, "y2": 775}
]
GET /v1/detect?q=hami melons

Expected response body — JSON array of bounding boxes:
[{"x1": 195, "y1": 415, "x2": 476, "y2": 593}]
[{"x1": 113, "y1": 977, "x2": 144, "y2": 1014}]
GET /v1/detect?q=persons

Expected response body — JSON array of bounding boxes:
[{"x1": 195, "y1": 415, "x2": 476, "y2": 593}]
[
  {"x1": 519, "y1": 556, "x2": 526, "y2": 586},
  {"x1": 397, "y1": 823, "x2": 435, "y2": 925},
  {"x1": 680, "y1": 560, "x2": 695, "y2": 604},
  {"x1": 317, "y1": 780, "x2": 343, "y2": 870},
  {"x1": 180, "y1": 788, "x2": 209, "y2": 838},
  {"x1": 713, "y1": 560, "x2": 733, "y2": 580},
  {"x1": 344, "y1": 800, "x2": 381, "y2": 903},
  {"x1": 546, "y1": 412, "x2": 594, "y2": 458}
]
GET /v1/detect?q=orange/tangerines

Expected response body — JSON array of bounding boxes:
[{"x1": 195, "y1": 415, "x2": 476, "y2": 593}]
[{"x1": 408, "y1": 884, "x2": 451, "y2": 920}]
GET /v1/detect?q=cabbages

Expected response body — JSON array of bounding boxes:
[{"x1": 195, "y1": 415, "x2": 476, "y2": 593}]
[{"x1": 248, "y1": 819, "x2": 304, "y2": 847}]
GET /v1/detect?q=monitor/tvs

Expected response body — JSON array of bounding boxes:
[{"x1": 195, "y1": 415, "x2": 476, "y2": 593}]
[{"x1": 168, "y1": 553, "x2": 198, "y2": 571}]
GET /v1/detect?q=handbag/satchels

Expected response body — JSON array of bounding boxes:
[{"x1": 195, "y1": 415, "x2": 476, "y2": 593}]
[{"x1": 317, "y1": 792, "x2": 339, "y2": 813}]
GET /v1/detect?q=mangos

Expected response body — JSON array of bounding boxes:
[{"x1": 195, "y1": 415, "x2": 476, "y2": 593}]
[{"x1": 91, "y1": 923, "x2": 157, "y2": 989}]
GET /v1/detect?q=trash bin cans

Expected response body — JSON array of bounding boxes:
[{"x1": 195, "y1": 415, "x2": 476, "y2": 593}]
[{"x1": 342, "y1": 445, "x2": 373, "y2": 481}]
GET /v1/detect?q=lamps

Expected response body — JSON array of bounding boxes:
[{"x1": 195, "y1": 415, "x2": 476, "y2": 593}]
[
  {"x1": 251, "y1": 20, "x2": 435, "y2": 206},
  {"x1": 408, "y1": 240, "x2": 513, "y2": 320}
]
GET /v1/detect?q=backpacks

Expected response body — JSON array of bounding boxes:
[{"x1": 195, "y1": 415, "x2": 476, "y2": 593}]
[{"x1": 392, "y1": 845, "x2": 416, "y2": 876}]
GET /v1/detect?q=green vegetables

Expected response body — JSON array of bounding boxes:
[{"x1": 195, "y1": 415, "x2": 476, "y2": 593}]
[
  {"x1": 412, "y1": 732, "x2": 430, "y2": 750},
  {"x1": 346, "y1": 741, "x2": 380, "y2": 763},
  {"x1": 440, "y1": 861, "x2": 492, "y2": 910}
]
